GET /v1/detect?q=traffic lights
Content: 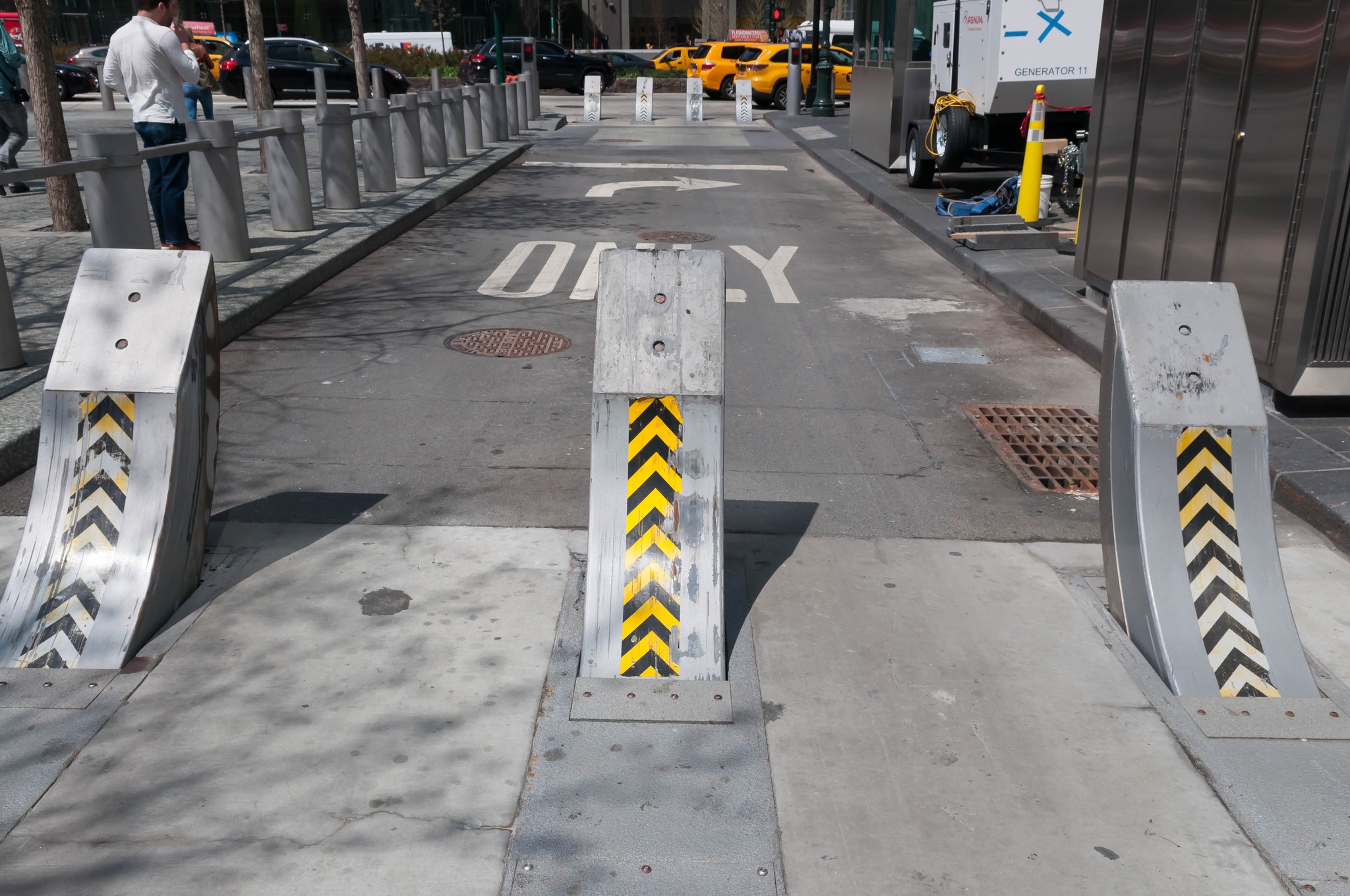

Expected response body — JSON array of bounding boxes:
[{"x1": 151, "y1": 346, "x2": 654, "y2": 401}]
[{"x1": 772, "y1": 8, "x2": 785, "y2": 22}]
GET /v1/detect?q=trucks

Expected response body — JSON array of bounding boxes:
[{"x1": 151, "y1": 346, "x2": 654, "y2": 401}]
[{"x1": 905, "y1": 0, "x2": 1104, "y2": 188}]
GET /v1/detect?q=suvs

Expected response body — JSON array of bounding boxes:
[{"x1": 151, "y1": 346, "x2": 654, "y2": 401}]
[
  {"x1": 686, "y1": 29, "x2": 773, "y2": 101},
  {"x1": 467, "y1": 37, "x2": 617, "y2": 95},
  {"x1": 217, "y1": 38, "x2": 410, "y2": 106},
  {"x1": 169, "y1": 21, "x2": 242, "y2": 92},
  {"x1": 64, "y1": 46, "x2": 109, "y2": 77},
  {"x1": 460, "y1": 38, "x2": 491, "y2": 87},
  {"x1": 731, "y1": 28, "x2": 855, "y2": 111}
]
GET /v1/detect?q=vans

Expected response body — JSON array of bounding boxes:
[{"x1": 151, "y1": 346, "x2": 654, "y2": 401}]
[{"x1": 797, "y1": 20, "x2": 924, "y2": 62}]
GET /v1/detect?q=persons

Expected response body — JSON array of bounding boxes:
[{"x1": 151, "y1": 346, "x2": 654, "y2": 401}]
[
  {"x1": 178, "y1": 24, "x2": 216, "y2": 122},
  {"x1": 103, "y1": 0, "x2": 201, "y2": 251},
  {"x1": 787, "y1": 29, "x2": 806, "y2": 43},
  {"x1": 0, "y1": 16, "x2": 30, "y2": 196}
]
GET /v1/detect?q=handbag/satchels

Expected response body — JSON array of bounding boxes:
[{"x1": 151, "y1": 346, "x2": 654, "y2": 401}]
[
  {"x1": 197, "y1": 63, "x2": 220, "y2": 91},
  {"x1": 10, "y1": 86, "x2": 30, "y2": 103}
]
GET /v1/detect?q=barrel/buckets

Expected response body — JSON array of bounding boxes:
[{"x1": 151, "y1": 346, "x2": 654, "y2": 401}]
[{"x1": 1017, "y1": 174, "x2": 1054, "y2": 219}]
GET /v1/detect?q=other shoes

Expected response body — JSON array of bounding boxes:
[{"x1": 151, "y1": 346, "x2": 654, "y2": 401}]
[
  {"x1": 161, "y1": 244, "x2": 169, "y2": 250},
  {"x1": 0, "y1": 185, "x2": 6, "y2": 195},
  {"x1": 169, "y1": 242, "x2": 200, "y2": 251},
  {"x1": 9, "y1": 184, "x2": 29, "y2": 193}
]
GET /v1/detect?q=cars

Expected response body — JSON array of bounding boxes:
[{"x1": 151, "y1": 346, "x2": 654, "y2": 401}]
[
  {"x1": 53, "y1": 63, "x2": 99, "y2": 101},
  {"x1": 646, "y1": 47, "x2": 700, "y2": 71},
  {"x1": 588, "y1": 52, "x2": 656, "y2": 70}
]
